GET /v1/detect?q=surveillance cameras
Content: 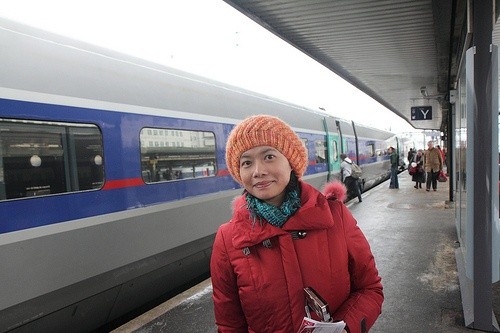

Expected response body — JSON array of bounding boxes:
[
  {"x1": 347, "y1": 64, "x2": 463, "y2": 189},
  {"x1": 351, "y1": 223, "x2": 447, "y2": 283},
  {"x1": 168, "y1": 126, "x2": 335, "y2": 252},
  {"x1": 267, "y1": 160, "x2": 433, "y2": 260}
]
[{"x1": 421, "y1": 89, "x2": 428, "y2": 97}]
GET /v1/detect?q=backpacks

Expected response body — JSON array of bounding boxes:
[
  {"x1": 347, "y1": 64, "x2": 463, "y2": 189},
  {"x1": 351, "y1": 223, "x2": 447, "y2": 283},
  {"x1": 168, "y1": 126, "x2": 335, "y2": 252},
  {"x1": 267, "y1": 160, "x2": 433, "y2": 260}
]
[{"x1": 344, "y1": 159, "x2": 361, "y2": 179}]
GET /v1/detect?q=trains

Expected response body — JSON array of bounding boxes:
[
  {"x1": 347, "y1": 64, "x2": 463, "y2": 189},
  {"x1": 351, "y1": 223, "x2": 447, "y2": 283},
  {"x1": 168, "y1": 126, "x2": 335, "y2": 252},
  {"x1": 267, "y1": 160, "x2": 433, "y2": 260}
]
[{"x1": 0, "y1": 18, "x2": 407, "y2": 333}]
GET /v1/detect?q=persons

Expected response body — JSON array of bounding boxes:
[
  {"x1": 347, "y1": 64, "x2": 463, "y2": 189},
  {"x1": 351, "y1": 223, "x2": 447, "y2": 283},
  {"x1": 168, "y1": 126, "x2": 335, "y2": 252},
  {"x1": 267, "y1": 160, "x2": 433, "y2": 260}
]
[
  {"x1": 407, "y1": 141, "x2": 447, "y2": 191},
  {"x1": 144, "y1": 154, "x2": 195, "y2": 183},
  {"x1": 456, "y1": 140, "x2": 467, "y2": 191},
  {"x1": 210, "y1": 114, "x2": 385, "y2": 333},
  {"x1": 339, "y1": 153, "x2": 362, "y2": 203},
  {"x1": 387, "y1": 146, "x2": 399, "y2": 188}
]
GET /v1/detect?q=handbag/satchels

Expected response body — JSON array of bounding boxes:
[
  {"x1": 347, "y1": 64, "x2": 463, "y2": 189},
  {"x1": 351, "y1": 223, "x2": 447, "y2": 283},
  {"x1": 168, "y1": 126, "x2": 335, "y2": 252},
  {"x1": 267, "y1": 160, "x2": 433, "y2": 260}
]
[
  {"x1": 409, "y1": 165, "x2": 416, "y2": 175},
  {"x1": 437, "y1": 170, "x2": 447, "y2": 183}
]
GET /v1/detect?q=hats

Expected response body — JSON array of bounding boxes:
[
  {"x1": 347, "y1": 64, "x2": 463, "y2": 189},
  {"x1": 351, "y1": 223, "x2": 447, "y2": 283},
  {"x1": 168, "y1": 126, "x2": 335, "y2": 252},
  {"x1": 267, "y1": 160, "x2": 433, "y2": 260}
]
[{"x1": 224, "y1": 114, "x2": 307, "y2": 188}]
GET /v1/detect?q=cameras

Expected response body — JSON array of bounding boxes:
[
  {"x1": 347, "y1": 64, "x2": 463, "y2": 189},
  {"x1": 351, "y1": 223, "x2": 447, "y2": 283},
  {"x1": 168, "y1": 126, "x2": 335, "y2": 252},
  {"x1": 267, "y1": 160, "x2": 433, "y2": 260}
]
[{"x1": 304, "y1": 288, "x2": 330, "y2": 321}]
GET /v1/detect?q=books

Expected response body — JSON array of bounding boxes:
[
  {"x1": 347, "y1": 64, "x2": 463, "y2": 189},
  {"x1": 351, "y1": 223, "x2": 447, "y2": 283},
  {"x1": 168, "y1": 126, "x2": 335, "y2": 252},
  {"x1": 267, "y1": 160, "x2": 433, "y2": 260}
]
[{"x1": 297, "y1": 316, "x2": 346, "y2": 333}]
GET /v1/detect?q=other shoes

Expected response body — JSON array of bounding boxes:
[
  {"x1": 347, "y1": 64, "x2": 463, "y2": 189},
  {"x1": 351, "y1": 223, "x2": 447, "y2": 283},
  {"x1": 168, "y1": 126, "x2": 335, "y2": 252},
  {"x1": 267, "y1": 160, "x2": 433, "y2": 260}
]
[
  {"x1": 426, "y1": 188, "x2": 430, "y2": 191},
  {"x1": 419, "y1": 184, "x2": 421, "y2": 188},
  {"x1": 359, "y1": 198, "x2": 362, "y2": 201},
  {"x1": 414, "y1": 185, "x2": 418, "y2": 189},
  {"x1": 434, "y1": 188, "x2": 437, "y2": 191}
]
